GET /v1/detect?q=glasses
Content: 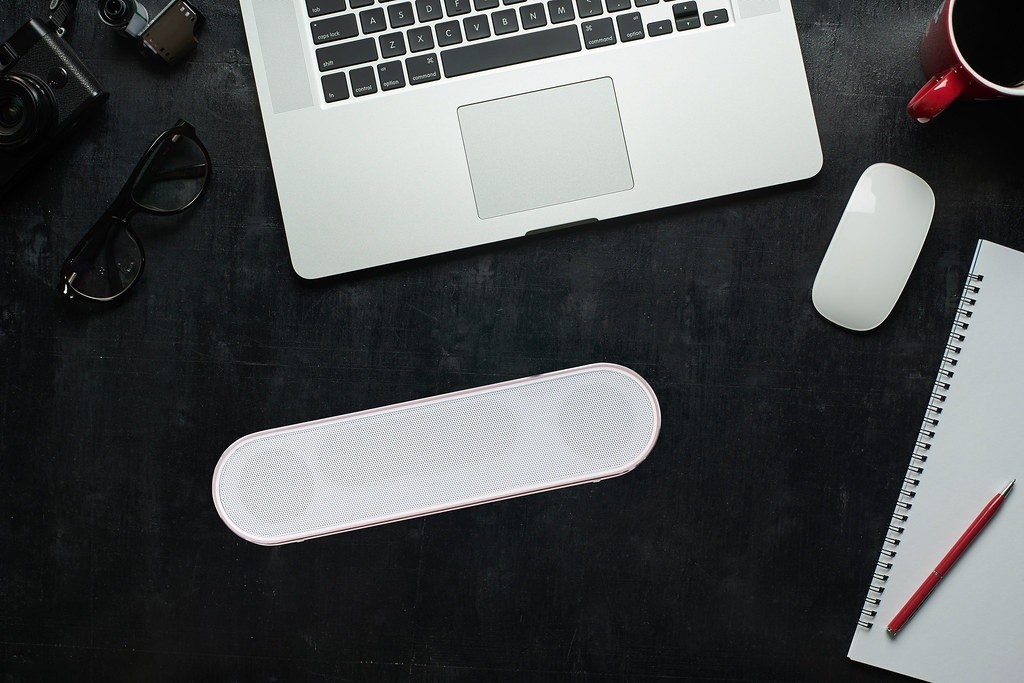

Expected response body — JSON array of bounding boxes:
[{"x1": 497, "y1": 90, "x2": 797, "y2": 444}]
[{"x1": 59, "y1": 119, "x2": 211, "y2": 301}]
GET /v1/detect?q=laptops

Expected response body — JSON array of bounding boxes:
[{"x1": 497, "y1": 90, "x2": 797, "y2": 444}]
[{"x1": 239, "y1": 0, "x2": 824, "y2": 279}]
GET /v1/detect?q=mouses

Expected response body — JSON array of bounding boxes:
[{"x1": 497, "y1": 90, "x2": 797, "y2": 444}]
[{"x1": 811, "y1": 163, "x2": 936, "y2": 331}]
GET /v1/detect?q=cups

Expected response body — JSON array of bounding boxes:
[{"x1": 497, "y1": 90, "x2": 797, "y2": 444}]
[{"x1": 906, "y1": 0, "x2": 1024, "y2": 123}]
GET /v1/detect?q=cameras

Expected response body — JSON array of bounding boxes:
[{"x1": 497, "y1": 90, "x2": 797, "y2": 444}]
[{"x1": 0, "y1": 16, "x2": 110, "y2": 178}]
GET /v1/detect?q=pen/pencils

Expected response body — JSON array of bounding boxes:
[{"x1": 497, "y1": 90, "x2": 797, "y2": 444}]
[{"x1": 886, "y1": 478, "x2": 1017, "y2": 639}]
